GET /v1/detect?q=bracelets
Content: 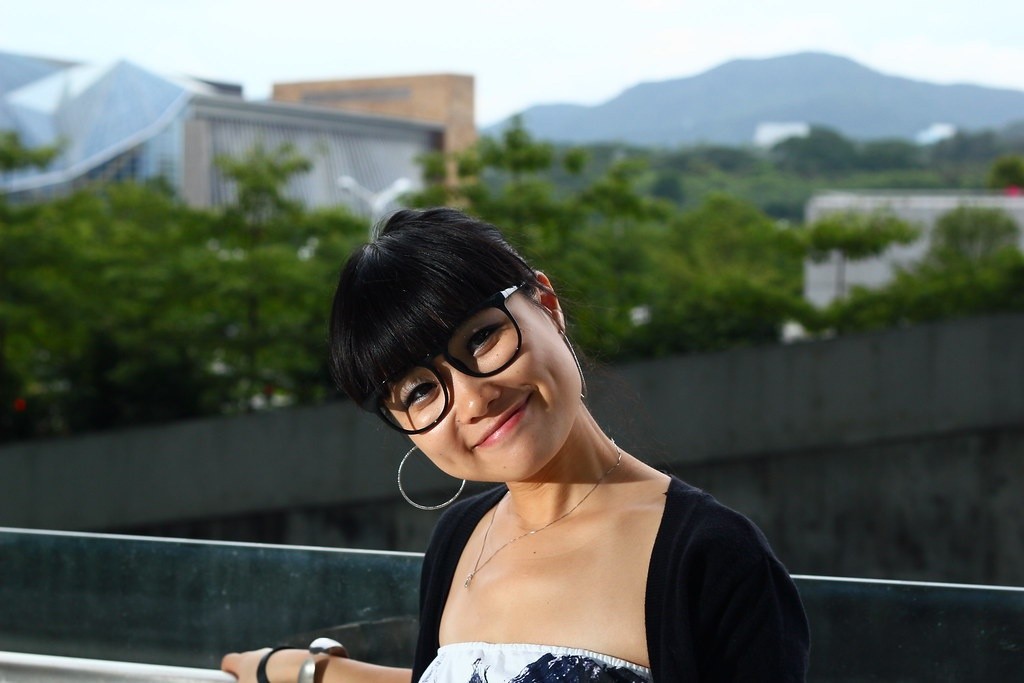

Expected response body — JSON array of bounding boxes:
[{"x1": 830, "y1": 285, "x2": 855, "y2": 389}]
[
  {"x1": 297, "y1": 637, "x2": 351, "y2": 683},
  {"x1": 255, "y1": 646, "x2": 298, "y2": 683}
]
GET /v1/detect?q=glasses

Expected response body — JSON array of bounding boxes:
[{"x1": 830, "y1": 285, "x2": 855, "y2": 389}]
[{"x1": 365, "y1": 277, "x2": 536, "y2": 435}]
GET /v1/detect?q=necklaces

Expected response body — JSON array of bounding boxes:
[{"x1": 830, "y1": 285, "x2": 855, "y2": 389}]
[{"x1": 463, "y1": 443, "x2": 622, "y2": 588}]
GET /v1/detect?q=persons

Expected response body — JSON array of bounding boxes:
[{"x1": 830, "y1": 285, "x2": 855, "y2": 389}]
[{"x1": 220, "y1": 207, "x2": 811, "y2": 683}]
